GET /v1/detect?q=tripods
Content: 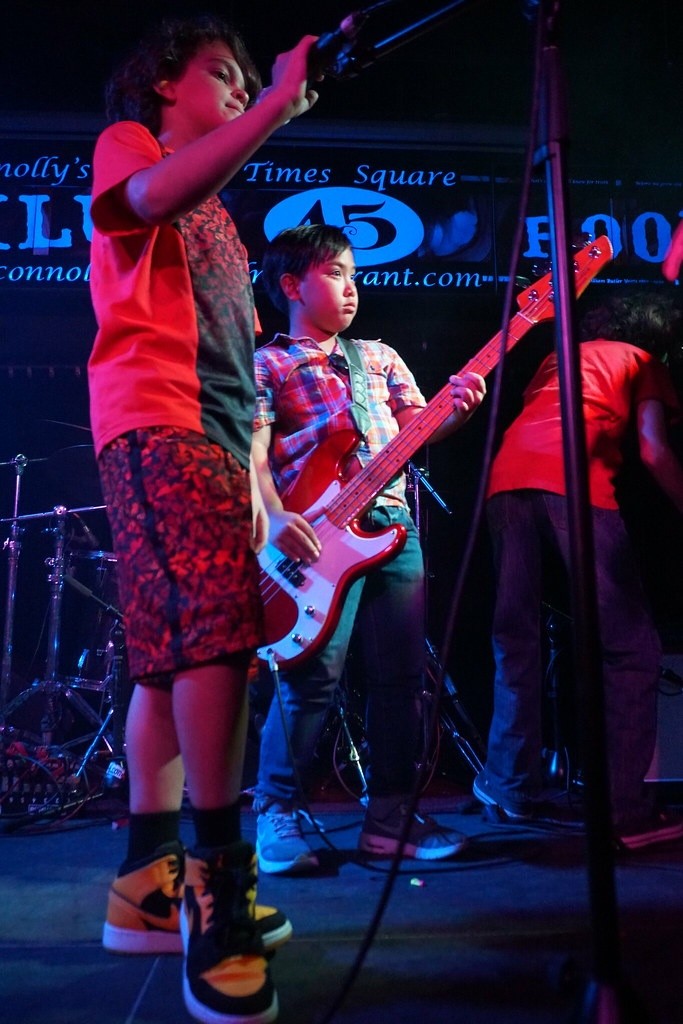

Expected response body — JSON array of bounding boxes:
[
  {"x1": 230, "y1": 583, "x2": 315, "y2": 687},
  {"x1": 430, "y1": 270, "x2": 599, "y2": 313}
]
[
  {"x1": 406, "y1": 462, "x2": 486, "y2": 785},
  {"x1": 0, "y1": 455, "x2": 131, "y2": 836}
]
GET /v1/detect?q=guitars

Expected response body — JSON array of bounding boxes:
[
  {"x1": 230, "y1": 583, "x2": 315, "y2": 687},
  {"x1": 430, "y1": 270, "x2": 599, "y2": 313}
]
[{"x1": 253, "y1": 230, "x2": 615, "y2": 671}]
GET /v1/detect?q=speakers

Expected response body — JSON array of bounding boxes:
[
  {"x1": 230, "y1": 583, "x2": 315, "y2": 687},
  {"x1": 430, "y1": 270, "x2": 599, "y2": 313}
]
[{"x1": 640, "y1": 649, "x2": 683, "y2": 794}]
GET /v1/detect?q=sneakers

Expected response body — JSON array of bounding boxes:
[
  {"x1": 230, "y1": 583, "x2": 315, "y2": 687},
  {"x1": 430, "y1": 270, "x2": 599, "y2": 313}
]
[
  {"x1": 256, "y1": 809, "x2": 326, "y2": 873},
  {"x1": 473, "y1": 771, "x2": 534, "y2": 820},
  {"x1": 103, "y1": 841, "x2": 294, "y2": 955},
  {"x1": 359, "y1": 805, "x2": 470, "y2": 861},
  {"x1": 615, "y1": 806, "x2": 683, "y2": 848},
  {"x1": 180, "y1": 846, "x2": 280, "y2": 1024}
]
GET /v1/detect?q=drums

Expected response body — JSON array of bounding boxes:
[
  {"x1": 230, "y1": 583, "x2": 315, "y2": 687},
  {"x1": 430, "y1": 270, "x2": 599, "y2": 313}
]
[{"x1": 58, "y1": 548, "x2": 127, "y2": 694}]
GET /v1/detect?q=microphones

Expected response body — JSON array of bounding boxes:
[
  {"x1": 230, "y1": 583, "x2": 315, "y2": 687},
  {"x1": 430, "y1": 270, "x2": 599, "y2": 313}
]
[
  {"x1": 661, "y1": 669, "x2": 683, "y2": 688},
  {"x1": 255, "y1": 11, "x2": 370, "y2": 127}
]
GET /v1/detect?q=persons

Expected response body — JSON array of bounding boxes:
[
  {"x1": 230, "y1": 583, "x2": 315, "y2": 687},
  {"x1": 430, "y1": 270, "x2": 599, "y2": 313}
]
[
  {"x1": 253, "y1": 224, "x2": 488, "y2": 872},
  {"x1": 87, "y1": 18, "x2": 321, "y2": 1024},
  {"x1": 473, "y1": 292, "x2": 683, "y2": 849}
]
